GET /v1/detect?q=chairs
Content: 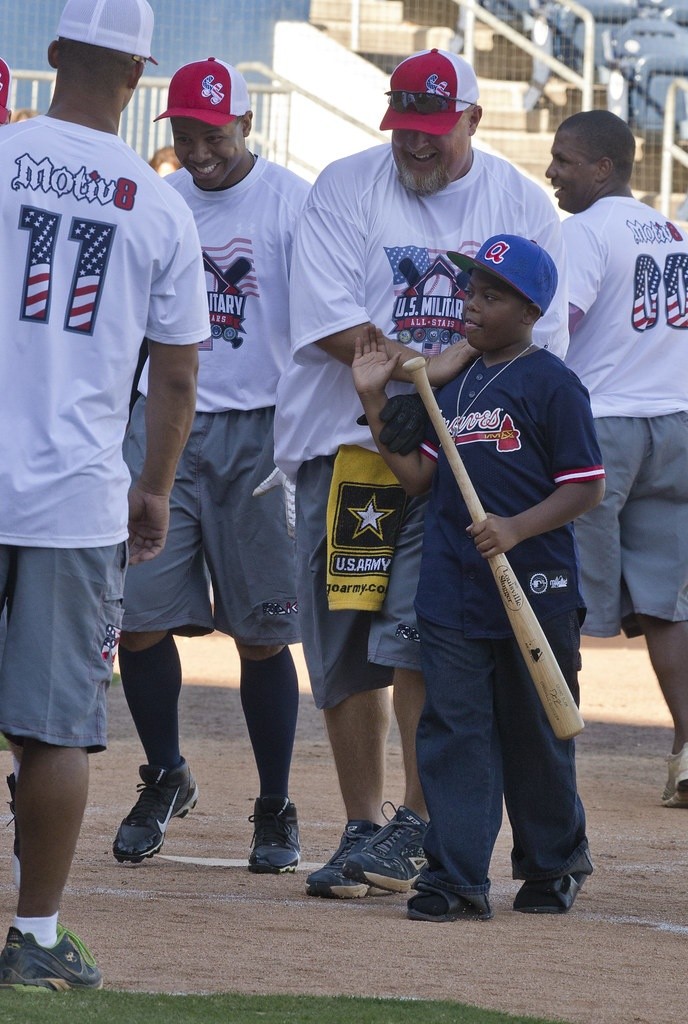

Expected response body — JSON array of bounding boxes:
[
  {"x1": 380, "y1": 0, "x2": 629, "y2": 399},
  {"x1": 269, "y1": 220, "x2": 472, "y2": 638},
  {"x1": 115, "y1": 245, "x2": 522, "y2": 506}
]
[{"x1": 476, "y1": 0, "x2": 688, "y2": 226}]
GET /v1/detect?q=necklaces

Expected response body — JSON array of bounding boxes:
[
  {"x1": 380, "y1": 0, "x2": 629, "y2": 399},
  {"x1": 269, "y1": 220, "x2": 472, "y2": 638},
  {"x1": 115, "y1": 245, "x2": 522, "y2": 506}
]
[{"x1": 452, "y1": 344, "x2": 534, "y2": 438}]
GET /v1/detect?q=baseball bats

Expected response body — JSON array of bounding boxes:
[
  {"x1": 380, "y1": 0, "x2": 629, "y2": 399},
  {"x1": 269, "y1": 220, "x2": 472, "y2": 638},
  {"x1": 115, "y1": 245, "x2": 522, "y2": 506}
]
[{"x1": 401, "y1": 357, "x2": 585, "y2": 741}]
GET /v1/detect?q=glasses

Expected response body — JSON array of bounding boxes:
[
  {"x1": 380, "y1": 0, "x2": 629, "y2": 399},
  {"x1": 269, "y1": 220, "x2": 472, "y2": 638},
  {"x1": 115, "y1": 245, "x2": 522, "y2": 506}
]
[{"x1": 383, "y1": 86, "x2": 478, "y2": 116}]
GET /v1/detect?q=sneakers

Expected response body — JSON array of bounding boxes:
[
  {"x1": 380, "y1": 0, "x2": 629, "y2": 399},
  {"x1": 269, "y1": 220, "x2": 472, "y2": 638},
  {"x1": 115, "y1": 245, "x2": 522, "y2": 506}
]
[
  {"x1": 248, "y1": 795, "x2": 302, "y2": 875},
  {"x1": 660, "y1": 740, "x2": 688, "y2": 809},
  {"x1": 5, "y1": 771, "x2": 23, "y2": 891},
  {"x1": 0, "y1": 922, "x2": 104, "y2": 993},
  {"x1": 111, "y1": 756, "x2": 197, "y2": 865},
  {"x1": 341, "y1": 804, "x2": 437, "y2": 893},
  {"x1": 305, "y1": 819, "x2": 393, "y2": 899}
]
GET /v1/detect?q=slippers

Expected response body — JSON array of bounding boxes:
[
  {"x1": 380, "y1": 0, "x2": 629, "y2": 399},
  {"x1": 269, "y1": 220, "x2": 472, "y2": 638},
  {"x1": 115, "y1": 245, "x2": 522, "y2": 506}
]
[
  {"x1": 407, "y1": 888, "x2": 493, "y2": 922},
  {"x1": 513, "y1": 868, "x2": 587, "y2": 915}
]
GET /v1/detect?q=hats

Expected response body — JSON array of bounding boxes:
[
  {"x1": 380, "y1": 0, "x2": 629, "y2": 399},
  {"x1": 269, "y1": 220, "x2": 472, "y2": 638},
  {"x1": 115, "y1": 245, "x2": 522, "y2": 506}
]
[
  {"x1": 0, "y1": 60, "x2": 12, "y2": 124},
  {"x1": 379, "y1": 50, "x2": 481, "y2": 136},
  {"x1": 446, "y1": 233, "x2": 558, "y2": 316},
  {"x1": 152, "y1": 57, "x2": 250, "y2": 127},
  {"x1": 56, "y1": 0, "x2": 159, "y2": 70}
]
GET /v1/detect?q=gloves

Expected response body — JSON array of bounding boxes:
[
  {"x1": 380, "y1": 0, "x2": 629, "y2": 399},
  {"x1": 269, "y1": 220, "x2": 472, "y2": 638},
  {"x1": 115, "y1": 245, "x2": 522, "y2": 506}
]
[{"x1": 357, "y1": 393, "x2": 431, "y2": 456}]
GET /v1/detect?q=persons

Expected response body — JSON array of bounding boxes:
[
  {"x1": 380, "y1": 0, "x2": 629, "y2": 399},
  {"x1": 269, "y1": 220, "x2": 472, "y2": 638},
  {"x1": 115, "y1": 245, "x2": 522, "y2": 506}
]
[
  {"x1": 0, "y1": 0, "x2": 212, "y2": 993},
  {"x1": 111, "y1": 58, "x2": 314, "y2": 873},
  {"x1": 351, "y1": 234, "x2": 605, "y2": 921},
  {"x1": 272, "y1": 50, "x2": 571, "y2": 899},
  {"x1": 545, "y1": 109, "x2": 688, "y2": 806}
]
